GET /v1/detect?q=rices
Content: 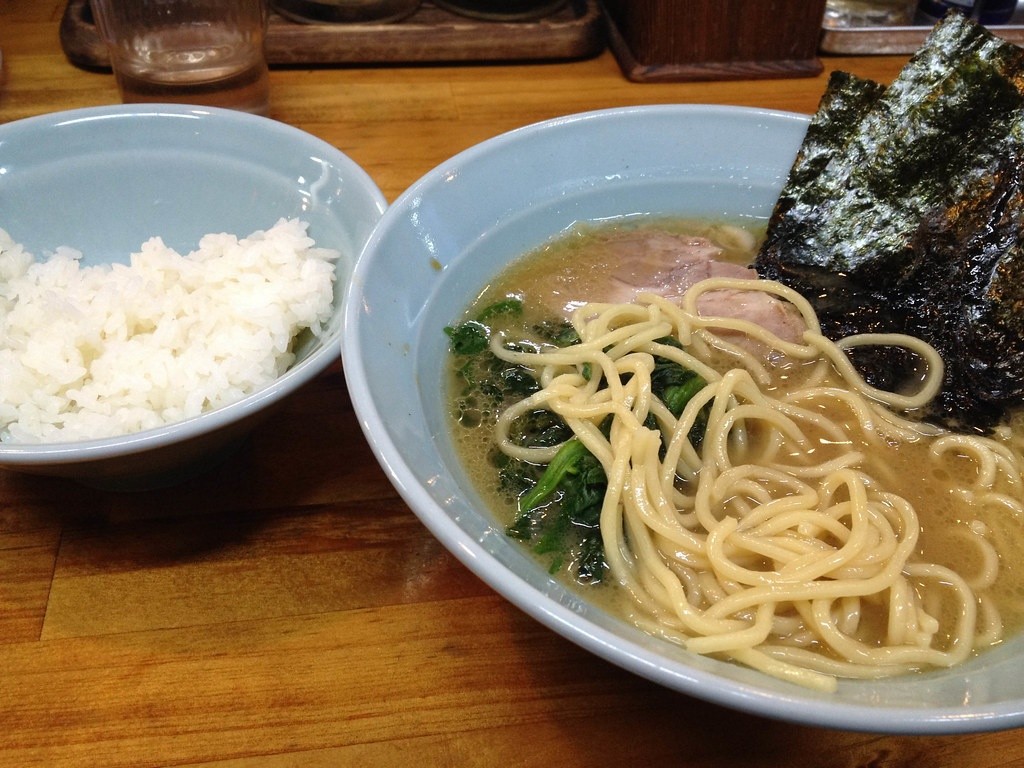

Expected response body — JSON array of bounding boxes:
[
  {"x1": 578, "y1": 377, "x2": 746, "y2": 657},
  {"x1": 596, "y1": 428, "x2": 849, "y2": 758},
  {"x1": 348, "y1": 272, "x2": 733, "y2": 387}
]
[{"x1": 1, "y1": 218, "x2": 340, "y2": 445}]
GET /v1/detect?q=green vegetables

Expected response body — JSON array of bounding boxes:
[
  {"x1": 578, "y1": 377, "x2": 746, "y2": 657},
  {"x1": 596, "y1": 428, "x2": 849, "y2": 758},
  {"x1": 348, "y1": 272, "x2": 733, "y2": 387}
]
[{"x1": 444, "y1": 300, "x2": 713, "y2": 581}]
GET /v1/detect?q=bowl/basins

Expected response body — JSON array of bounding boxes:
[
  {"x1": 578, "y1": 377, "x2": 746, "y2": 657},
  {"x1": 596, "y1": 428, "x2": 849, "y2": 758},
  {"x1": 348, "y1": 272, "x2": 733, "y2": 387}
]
[
  {"x1": 337, "y1": 104, "x2": 1024, "y2": 735},
  {"x1": 0, "y1": 102, "x2": 390, "y2": 469}
]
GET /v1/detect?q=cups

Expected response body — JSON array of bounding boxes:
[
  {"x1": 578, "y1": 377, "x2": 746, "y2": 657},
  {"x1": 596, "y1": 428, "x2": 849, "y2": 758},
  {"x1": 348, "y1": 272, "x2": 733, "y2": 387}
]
[{"x1": 91, "y1": 0, "x2": 271, "y2": 120}]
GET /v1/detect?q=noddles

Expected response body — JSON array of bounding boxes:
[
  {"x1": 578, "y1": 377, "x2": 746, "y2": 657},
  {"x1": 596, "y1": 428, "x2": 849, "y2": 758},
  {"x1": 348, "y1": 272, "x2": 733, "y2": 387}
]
[{"x1": 489, "y1": 276, "x2": 1023, "y2": 691}]
